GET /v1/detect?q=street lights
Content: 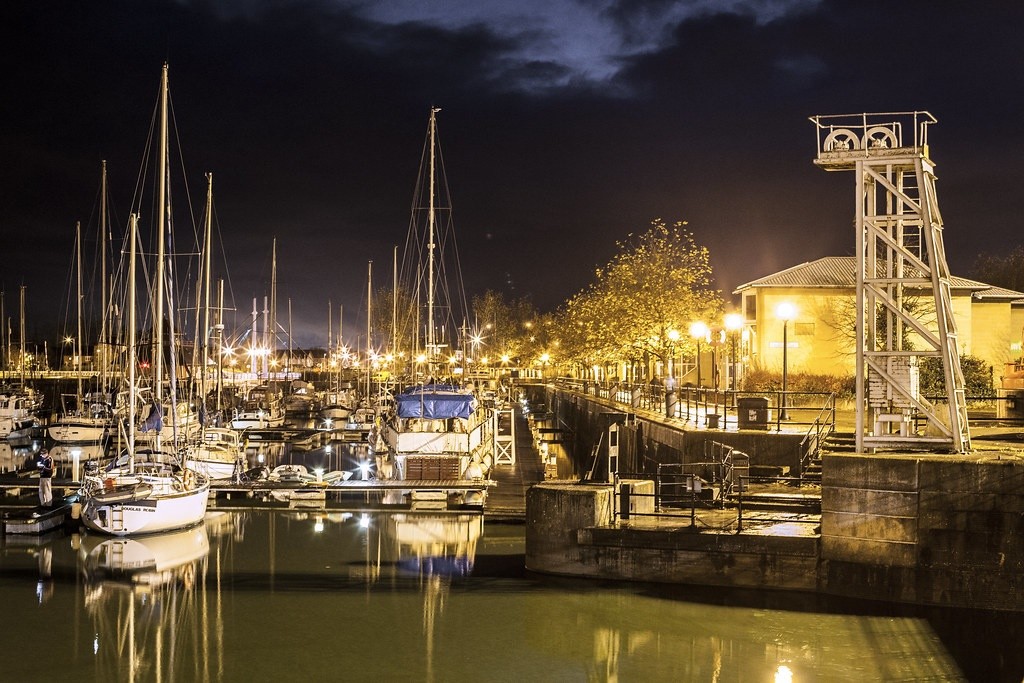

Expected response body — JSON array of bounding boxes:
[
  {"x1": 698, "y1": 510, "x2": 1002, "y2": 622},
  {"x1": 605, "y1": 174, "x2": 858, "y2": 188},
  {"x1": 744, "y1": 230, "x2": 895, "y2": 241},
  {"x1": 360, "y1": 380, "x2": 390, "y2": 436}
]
[
  {"x1": 772, "y1": 302, "x2": 795, "y2": 421},
  {"x1": 690, "y1": 322, "x2": 706, "y2": 402},
  {"x1": 65, "y1": 338, "x2": 76, "y2": 371},
  {"x1": 669, "y1": 329, "x2": 677, "y2": 385},
  {"x1": 726, "y1": 312, "x2": 740, "y2": 412}
]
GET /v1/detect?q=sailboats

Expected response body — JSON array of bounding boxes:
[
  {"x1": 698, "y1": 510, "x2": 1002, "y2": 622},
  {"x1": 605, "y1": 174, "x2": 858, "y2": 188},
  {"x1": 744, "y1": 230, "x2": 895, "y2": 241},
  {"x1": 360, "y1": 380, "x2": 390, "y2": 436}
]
[
  {"x1": 212, "y1": 507, "x2": 485, "y2": 683},
  {"x1": 0, "y1": 63, "x2": 494, "y2": 538},
  {"x1": 64, "y1": 513, "x2": 237, "y2": 683}
]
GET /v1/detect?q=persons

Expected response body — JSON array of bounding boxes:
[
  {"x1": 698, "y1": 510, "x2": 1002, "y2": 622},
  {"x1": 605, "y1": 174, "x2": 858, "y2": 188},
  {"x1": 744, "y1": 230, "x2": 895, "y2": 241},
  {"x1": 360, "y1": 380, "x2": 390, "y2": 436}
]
[{"x1": 37, "y1": 448, "x2": 54, "y2": 509}]
